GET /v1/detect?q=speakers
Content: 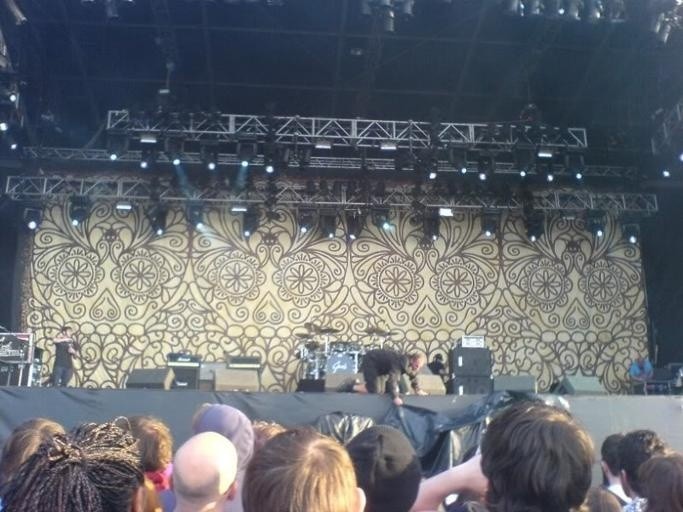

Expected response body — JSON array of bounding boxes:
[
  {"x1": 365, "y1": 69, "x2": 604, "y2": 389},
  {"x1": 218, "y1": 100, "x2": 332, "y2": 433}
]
[
  {"x1": 401, "y1": 374, "x2": 447, "y2": 395},
  {"x1": 323, "y1": 373, "x2": 365, "y2": 392},
  {"x1": 493, "y1": 375, "x2": 538, "y2": 396},
  {"x1": 229, "y1": 356, "x2": 261, "y2": 390},
  {"x1": 0, "y1": 333, "x2": 44, "y2": 387},
  {"x1": 167, "y1": 354, "x2": 199, "y2": 390},
  {"x1": 448, "y1": 377, "x2": 492, "y2": 396}
]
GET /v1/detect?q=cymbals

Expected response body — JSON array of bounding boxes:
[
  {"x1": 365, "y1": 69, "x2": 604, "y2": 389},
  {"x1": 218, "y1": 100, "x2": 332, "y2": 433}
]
[
  {"x1": 318, "y1": 329, "x2": 338, "y2": 333},
  {"x1": 367, "y1": 328, "x2": 386, "y2": 335},
  {"x1": 305, "y1": 323, "x2": 320, "y2": 334},
  {"x1": 296, "y1": 333, "x2": 313, "y2": 339}
]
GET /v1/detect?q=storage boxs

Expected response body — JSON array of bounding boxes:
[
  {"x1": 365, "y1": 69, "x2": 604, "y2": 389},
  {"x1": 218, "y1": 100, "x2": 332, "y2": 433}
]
[
  {"x1": 415, "y1": 375, "x2": 447, "y2": 395},
  {"x1": 495, "y1": 375, "x2": 536, "y2": 392},
  {"x1": 169, "y1": 366, "x2": 201, "y2": 390},
  {"x1": 445, "y1": 377, "x2": 495, "y2": 393},
  {"x1": 296, "y1": 379, "x2": 326, "y2": 392}
]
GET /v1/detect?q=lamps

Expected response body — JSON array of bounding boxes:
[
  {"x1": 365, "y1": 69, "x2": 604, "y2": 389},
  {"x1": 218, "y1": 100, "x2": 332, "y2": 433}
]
[
  {"x1": 508, "y1": 1, "x2": 682, "y2": 44},
  {"x1": 115, "y1": 201, "x2": 132, "y2": 212},
  {"x1": 145, "y1": 178, "x2": 169, "y2": 235},
  {"x1": 0, "y1": 2, "x2": 27, "y2": 152},
  {"x1": 68, "y1": 196, "x2": 91, "y2": 227},
  {"x1": 521, "y1": 205, "x2": 545, "y2": 243},
  {"x1": 158, "y1": 66, "x2": 170, "y2": 94},
  {"x1": 622, "y1": 224, "x2": 640, "y2": 244},
  {"x1": 419, "y1": 151, "x2": 586, "y2": 183},
  {"x1": 480, "y1": 208, "x2": 501, "y2": 237},
  {"x1": 23, "y1": 203, "x2": 42, "y2": 231},
  {"x1": 411, "y1": 201, "x2": 440, "y2": 242},
  {"x1": 298, "y1": 205, "x2": 388, "y2": 239},
  {"x1": 241, "y1": 205, "x2": 261, "y2": 238},
  {"x1": 584, "y1": 211, "x2": 607, "y2": 237},
  {"x1": 188, "y1": 206, "x2": 203, "y2": 229},
  {"x1": 107, "y1": 137, "x2": 281, "y2": 174},
  {"x1": 359, "y1": 2, "x2": 416, "y2": 41},
  {"x1": 662, "y1": 146, "x2": 682, "y2": 180}
]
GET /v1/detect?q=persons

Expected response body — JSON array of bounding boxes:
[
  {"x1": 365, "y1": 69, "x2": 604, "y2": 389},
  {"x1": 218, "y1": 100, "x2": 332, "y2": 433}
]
[
  {"x1": 406, "y1": 398, "x2": 622, "y2": 512},
  {"x1": 428, "y1": 353, "x2": 446, "y2": 379},
  {"x1": 52, "y1": 326, "x2": 83, "y2": 387},
  {"x1": 599, "y1": 429, "x2": 683, "y2": 512},
  {"x1": 169, "y1": 403, "x2": 422, "y2": 512},
  {"x1": 630, "y1": 355, "x2": 656, "y2": 396},
  {"x1": 338, "y1": 350, "x2": 429, "y2": 405},
  {"x1": 1, "y1": 416, "x2": 174, "y2": 512}
]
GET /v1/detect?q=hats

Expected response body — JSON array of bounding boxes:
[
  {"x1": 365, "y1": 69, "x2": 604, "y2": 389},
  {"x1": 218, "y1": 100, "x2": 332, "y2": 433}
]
[
  {"x1": 345, "y1": 425, "x2": 420, "y2": 511},
  {"x1": 194, "y1": 403, "x2": 254, "y2": 468}
]
